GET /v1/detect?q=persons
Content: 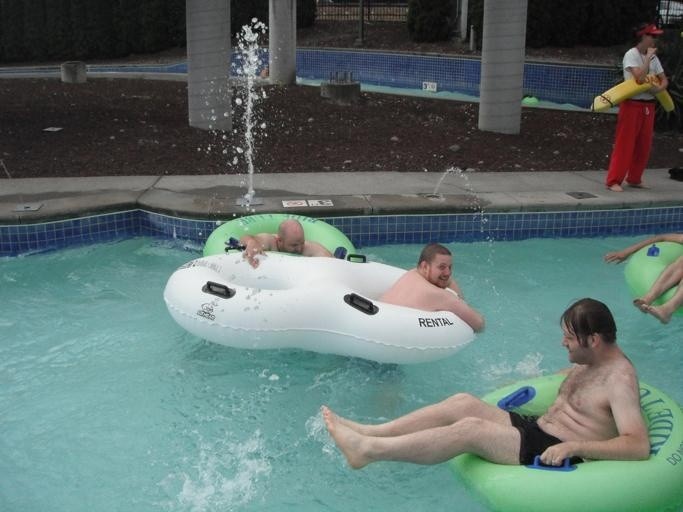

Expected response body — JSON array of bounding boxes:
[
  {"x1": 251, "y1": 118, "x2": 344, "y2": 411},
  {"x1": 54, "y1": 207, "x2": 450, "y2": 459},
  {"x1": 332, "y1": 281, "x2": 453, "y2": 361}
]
[
  {"x1": 238, "y1": 219, "x2": 338, "y2": 273},
  {"x1": 599, "y1": 20, "x2": 670, "y2": 193},
  {"x1": 320, "y1": 297, "x2": 653, "y2": 469},
  {"x1": 602, "y1": 231, "x2": 683, "y2": 325},
  {"x1": 378, "y1": 243, "x2": 486, "y2": 332}
]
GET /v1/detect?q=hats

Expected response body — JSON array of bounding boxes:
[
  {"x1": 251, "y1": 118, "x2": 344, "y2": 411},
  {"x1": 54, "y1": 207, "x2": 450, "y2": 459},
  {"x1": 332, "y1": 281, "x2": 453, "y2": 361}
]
[{"x1": 636, "y1": 25, "x2": 665, "y2": 36}]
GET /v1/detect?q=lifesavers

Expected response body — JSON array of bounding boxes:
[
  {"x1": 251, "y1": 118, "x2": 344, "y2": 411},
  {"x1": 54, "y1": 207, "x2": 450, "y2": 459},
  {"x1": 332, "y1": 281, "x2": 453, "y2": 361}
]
[
  {"x1": 445, "y1": 364, "x2": 682, "y2": 496},
  {"x1": 204, "y1": 212, "x2": 358, "y2": 261},
  {"x1": 623, "y1": 240, "x2": 683, "y2": 309},
  {"x1": 161, "y1": 250, "x2": 472, "y2": 367},
  {"x1": 522, "y1": 94, "x2": 539, "y2": 106}
]
